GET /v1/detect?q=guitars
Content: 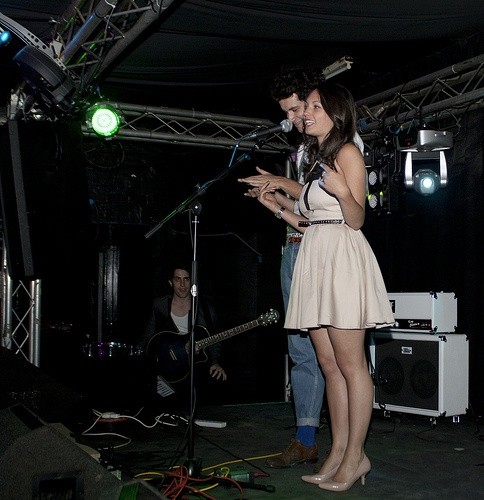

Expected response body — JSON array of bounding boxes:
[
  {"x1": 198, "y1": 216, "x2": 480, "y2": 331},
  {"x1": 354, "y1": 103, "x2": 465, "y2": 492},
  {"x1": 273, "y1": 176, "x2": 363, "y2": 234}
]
[{"x1": 145, "y1": 306, "x2": 281, "y2": 384}]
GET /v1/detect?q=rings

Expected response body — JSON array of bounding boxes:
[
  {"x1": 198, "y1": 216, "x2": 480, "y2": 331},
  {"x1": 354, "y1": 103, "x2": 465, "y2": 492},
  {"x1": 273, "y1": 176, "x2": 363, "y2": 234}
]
[{"x1": 321, "y1": 171, "x2": 327, "y2": 177}]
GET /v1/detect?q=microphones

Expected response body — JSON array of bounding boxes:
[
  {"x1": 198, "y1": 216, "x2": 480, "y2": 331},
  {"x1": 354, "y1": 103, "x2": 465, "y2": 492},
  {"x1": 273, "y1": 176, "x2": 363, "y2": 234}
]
[{"x1": 241, "y1": 119, "x2": 293, "y2": 140}]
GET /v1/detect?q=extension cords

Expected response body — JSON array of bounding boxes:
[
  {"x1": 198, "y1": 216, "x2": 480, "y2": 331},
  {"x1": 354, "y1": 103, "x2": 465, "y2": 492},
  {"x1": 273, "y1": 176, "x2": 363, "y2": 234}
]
[{"x1": 193, "y1": 419, "x2": 227, "y2": 428}]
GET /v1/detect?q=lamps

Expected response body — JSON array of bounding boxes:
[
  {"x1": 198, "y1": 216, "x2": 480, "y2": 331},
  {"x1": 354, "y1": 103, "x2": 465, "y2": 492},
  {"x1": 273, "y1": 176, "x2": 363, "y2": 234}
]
[
  {"x1": 365, "y1": 171, "x2": 389, "y2": 208},
  {"x1": 405, "y1": 151, "x2": 448, "y2": 195},
  {"x1": 90, "y1": 100, "x2": 120, "y2": 138}
]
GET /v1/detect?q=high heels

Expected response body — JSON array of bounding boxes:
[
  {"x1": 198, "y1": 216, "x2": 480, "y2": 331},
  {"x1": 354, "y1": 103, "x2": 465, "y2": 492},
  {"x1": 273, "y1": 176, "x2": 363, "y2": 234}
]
[{"x1": 301, "y1": 454, "x2": 371, "y2": 491}]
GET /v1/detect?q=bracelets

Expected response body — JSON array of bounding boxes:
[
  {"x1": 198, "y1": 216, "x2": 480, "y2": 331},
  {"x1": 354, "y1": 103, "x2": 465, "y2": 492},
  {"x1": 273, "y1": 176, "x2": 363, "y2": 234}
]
[{"x1": 274, "y1": 206, "x2": 286, "y2": 219}]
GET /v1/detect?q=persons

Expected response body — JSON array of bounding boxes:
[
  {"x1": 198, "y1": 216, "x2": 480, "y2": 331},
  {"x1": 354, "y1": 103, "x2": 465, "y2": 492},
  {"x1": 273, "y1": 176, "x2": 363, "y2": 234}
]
[
  {"x1": 138, "y1": 263, "x2": 230, "y2": 402},
  {"x1": 258, "y1": 82, "x2": 395, "y2": 491},
  {"x1": 237, "y1": 84, "x2": 365, "y2": 466}
]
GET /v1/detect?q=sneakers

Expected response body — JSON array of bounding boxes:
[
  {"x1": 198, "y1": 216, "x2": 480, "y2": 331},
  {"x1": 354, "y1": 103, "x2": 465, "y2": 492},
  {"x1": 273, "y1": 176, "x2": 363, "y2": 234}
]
[{"x1": 263, "y1": 439, "x2": 320, "y2": 468}]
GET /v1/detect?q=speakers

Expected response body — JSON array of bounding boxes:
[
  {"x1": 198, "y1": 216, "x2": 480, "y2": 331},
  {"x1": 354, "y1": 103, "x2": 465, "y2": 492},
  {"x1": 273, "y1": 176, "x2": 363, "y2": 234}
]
[
  {"x1": 362, "y1": 332, "x2": 469, "y2": 418},
  {"x1": 0, "y1": 403, "x2": 123, "y2": 500}
]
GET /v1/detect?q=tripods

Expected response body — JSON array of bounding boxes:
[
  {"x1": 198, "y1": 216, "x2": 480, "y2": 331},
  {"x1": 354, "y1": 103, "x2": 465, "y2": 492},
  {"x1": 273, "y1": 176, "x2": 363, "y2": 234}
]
[{"x1": 145, "y1": 141, "x2": 276, "y2": 500}]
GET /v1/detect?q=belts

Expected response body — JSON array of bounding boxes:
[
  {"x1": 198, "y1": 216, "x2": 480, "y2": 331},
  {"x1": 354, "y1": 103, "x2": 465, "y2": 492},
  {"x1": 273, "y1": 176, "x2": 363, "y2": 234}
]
[
  {"x1": 299, "y1": 219, "x2": 347, "y2": 227},
  {"x1": 288, "y1": 238, "x2": 301, "y2": 242}
]
